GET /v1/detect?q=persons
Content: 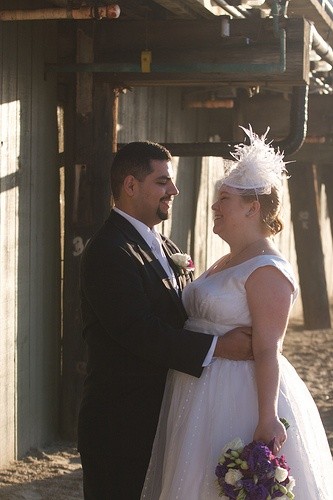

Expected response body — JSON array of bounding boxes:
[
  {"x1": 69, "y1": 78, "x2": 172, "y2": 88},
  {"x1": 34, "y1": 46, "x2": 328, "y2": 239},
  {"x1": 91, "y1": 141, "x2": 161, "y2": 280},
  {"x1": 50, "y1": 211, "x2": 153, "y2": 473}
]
[
  {"x1": 77, "y1": 140, "x2": 253, "y2": 500},
  {"x1": 140, "y1": 123, "x2": 333, "y2": 500}
]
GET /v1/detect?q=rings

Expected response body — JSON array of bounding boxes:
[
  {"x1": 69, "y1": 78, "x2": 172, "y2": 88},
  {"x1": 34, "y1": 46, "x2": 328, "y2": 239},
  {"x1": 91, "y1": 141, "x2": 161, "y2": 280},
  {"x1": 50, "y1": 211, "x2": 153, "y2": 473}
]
[{"x1": 280, "y1": 440, "x2": 285, "y2": 443}]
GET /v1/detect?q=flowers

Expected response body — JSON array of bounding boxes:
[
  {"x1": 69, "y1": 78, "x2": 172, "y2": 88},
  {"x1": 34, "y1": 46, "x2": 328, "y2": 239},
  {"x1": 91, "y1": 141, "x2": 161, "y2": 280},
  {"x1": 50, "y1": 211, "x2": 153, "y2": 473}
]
[
  {"x1": 170, "y1": 253, "x2": 196, "y2": 279},
  {"x1": 214, "y1": 418, "x2": 296, "y2": 500}
]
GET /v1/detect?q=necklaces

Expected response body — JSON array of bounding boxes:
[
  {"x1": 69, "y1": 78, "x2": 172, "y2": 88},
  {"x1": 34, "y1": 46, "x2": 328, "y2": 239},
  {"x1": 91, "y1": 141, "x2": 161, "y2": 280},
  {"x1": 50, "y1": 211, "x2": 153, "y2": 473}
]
[{"x1": 213, "y1": 238, "x2": 262, "y2": 269}]
